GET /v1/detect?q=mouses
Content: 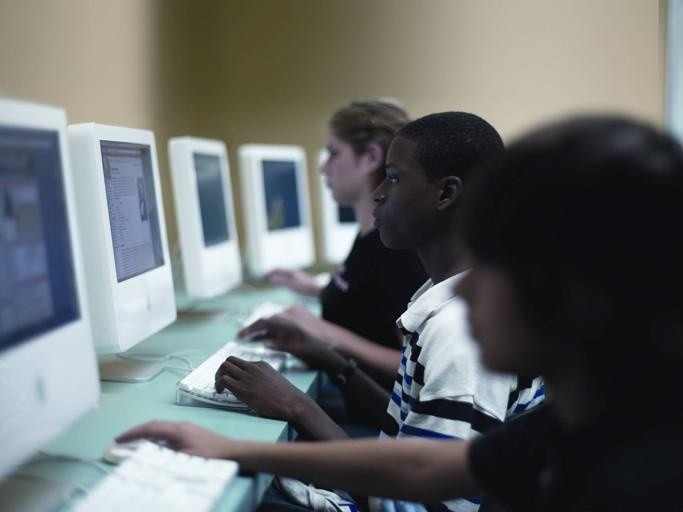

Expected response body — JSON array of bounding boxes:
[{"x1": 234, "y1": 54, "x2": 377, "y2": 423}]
[{"x1": 102, "y1": 436, "x2": 169, "y2": 463}]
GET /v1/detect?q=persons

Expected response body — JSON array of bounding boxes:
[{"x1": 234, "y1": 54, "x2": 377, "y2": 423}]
[
  {"x1": 214, "y1": 112, "x2": 546, "y2": 511},
  {"x1": 115, "y1": 110, "x2": 683, "y2": 511},
  {"x1": 266, "y1": 95, "x2": 429, "y2": 419}
]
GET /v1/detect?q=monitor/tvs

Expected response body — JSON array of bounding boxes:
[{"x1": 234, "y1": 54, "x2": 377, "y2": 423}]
[
  {"x1": 0, "y1": 97, "x2": 102, "y2": 482},
  {"x1": 166, "y1": 134, "x2": 243, "y2": 323},
  {"x1": 234, "y1": 141, "x2": 316, "y2": 287},
  {"x1": 311, "y1": 145, "x2": 362, "y2": 265},
  {"x1": 63, "y1": 120, "x2": 177, "y2": 385}
]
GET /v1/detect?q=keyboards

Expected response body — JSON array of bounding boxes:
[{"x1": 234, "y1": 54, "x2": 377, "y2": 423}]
[
  {"x1": 178, "y1": 339, "x2": 287, "y2": 419},
  {"x1": 241, "y1": 302, "x2": 284, "y2": 323},
  {"x1": 45, "y1": 435, "x2": 240, "y2": 512}
]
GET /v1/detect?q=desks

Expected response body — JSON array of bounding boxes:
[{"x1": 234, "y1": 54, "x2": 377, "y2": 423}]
[{"x1": 1, "y1": 260, "x2": 341, "y2": 512}]
